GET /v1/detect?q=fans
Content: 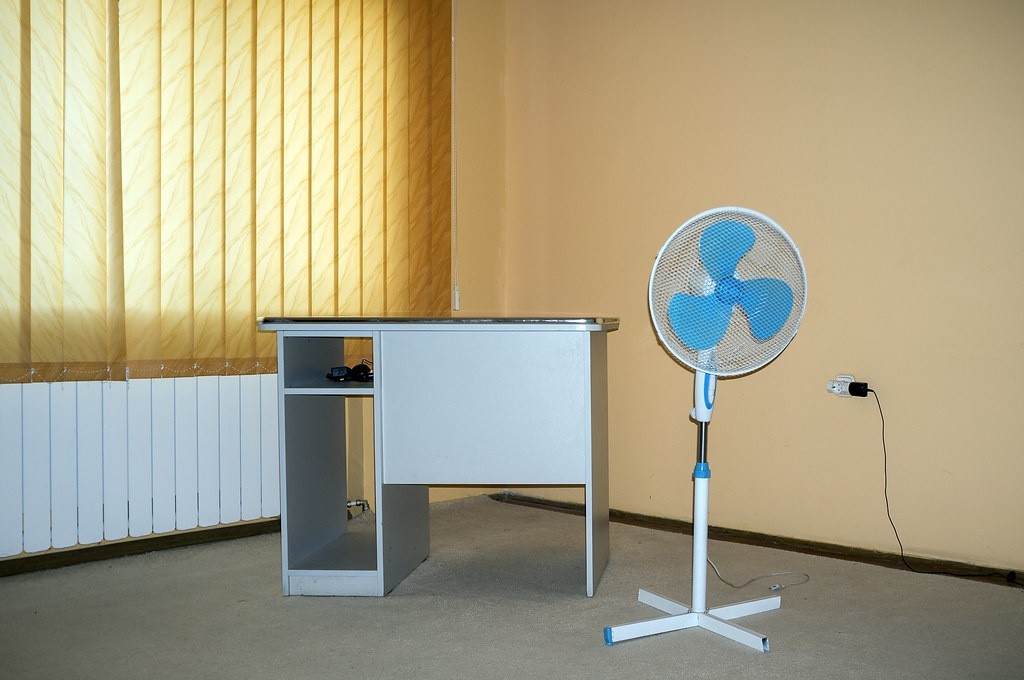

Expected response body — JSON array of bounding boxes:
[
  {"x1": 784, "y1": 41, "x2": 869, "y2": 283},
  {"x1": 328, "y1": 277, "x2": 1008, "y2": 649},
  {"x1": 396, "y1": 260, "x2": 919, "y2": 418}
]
[{"x1": 603, "y1": 208, "x2": 810, "y2": 652}]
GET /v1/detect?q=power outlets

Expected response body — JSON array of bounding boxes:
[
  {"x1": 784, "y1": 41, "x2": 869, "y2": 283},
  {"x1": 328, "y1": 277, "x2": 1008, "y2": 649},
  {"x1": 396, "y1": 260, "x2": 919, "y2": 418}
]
[{"x1": 829, "y1": 371, "x2": 856, "y2": 399}]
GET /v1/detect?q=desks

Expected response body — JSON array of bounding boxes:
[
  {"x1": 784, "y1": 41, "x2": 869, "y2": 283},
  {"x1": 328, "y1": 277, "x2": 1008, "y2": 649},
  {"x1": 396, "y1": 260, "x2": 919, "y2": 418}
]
[{"x1": 260, "y1": 316, "x2": 616, "y2": 596}]
